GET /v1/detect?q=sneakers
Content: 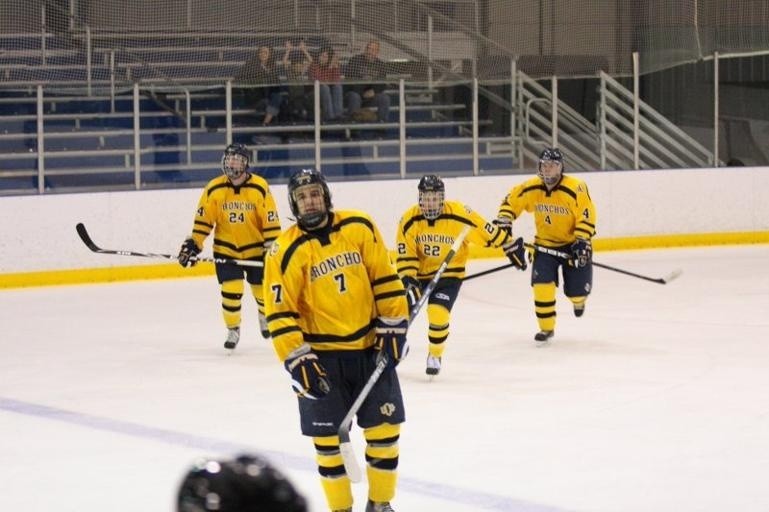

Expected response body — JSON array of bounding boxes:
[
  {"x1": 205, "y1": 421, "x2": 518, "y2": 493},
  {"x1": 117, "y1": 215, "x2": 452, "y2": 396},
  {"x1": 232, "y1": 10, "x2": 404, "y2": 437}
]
[
  {"x1": 574, "y1": 301, "x2": 584, "y2": 316},
  {"x1": 426, "y1": 353, "x2": 441, "y2": 375},
  {"x1": 224, "y1": 327, "x2": 240, "y2": 349},
  {"x1": 535, "y1": 330, "x2": 554, "y2": 341},
  {"x1": 257, "y1": 312, "x2": 271, "y2": 338},
  {"x1": 364, "y1": 499, "x2": 396, "y2": 512}
]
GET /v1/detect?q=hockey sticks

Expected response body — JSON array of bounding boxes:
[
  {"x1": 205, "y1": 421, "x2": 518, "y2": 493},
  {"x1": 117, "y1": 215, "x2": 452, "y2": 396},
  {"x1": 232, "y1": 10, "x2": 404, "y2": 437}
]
[
  {"x1": 339, "y1": 225, "x2": 472, "y2": 481},
  {"x1": 76, "y1": 222, "x2": 264, "y2": 268},
  {"x1": 524, "y1": 242, "x2": 684, "y2": 284}
]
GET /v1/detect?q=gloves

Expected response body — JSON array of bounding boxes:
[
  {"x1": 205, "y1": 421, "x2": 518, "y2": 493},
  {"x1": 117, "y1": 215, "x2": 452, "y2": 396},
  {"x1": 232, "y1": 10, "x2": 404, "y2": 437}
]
[
  {"x1": 283, "y1": 344, "x2": 334, "y2": 400},
  {"x1": 403, "y1": 277, "x2": 422, "y2": 309},
  {"x1": 502, "y1": 237, "x2": 527, "y2": 270},
  {"x1": 570, "y1": 238, "x2": 591, "y2": 268},
  {"x1": 373, "y1": 315, "x2": 410, "y2": 372},
  {"x1": 177, "y1": 239, "x2": 199, "y2": 267}
]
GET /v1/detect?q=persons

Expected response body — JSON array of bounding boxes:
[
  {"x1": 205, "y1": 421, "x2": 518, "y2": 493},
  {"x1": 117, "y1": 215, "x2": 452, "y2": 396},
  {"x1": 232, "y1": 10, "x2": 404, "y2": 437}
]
[
  {"x1": 175, "y1": 142, "x2": 284, "y2": 352},
  {"x1": 259, "y1": 164, "x2": 409, "y2": 511},
  {"x1": 234, "y1": 43, "x2": 283, "y2": 127},
  {"x1": 345, "y1": 38, "x2": 393, "y2": 141},
  {"x1": 308, "y1": 46, "x2": 344, "y2": 124},
  {"x1": 496, "y1": 147, "x2": 600, "y2": 344},
  {"x1": 278, "y1": 35, "x2": 315, "y2": 124},
  {"x1": 395, "y1": 172, "x2": 526, "y2": 378}
]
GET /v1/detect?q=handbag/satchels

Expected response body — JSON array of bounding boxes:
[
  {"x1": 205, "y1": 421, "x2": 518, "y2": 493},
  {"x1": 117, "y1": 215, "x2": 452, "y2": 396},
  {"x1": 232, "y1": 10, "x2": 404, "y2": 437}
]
[{"x1": 351, "y1": 108, "x2": 379, "y2": 122}]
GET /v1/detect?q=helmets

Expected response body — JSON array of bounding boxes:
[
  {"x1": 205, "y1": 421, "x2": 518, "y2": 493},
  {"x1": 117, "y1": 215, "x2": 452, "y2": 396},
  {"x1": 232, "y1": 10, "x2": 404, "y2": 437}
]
[
  {"x1": 538, "y1": 147, "x2": 564, "y2": 184},
  {"x1": 178, "y1": 455, "x2": 307, "y2": 511},
  {"x1": 221, "y1": 143, "x2": 250, "y2": 180},
  {"x1": 288, "y1": 169, "x2": 331, "y2": 226},
  {"x1": 418, "y1": 175, "x2": 445, "y2": 220}
]
